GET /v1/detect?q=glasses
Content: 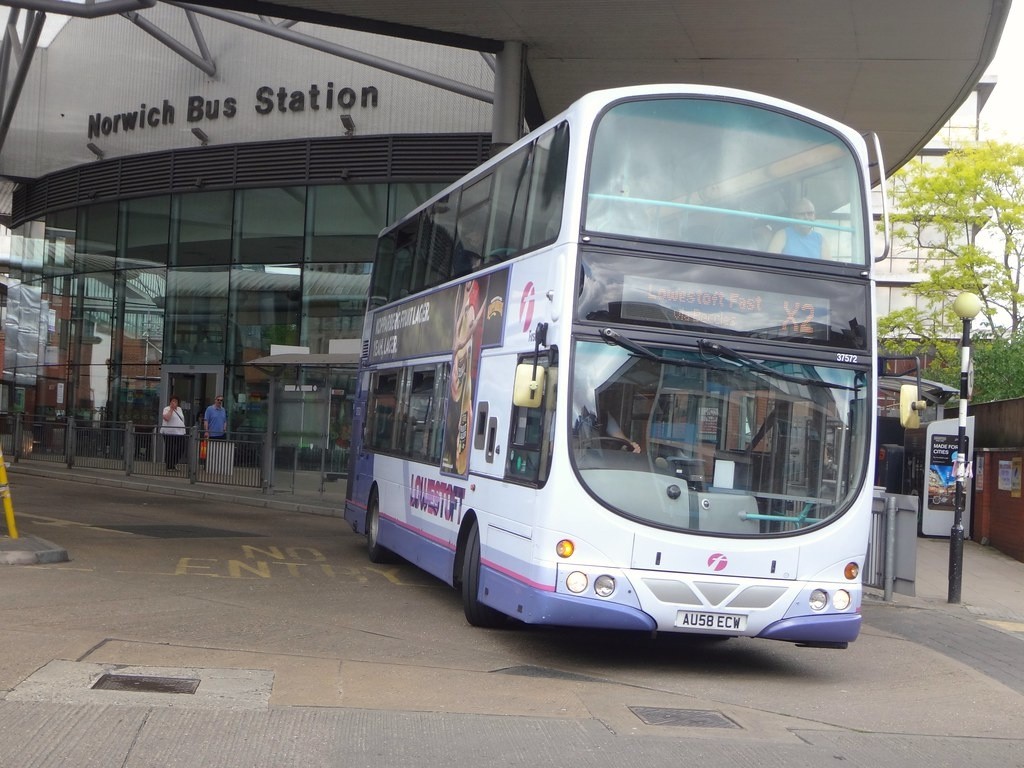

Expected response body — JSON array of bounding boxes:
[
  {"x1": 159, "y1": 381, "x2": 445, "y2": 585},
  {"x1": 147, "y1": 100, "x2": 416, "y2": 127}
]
[
  {"x1": 216, "y1": 398, "x2": 223, "y2": 401},
  {"x1": 796, "y1": 211, "x2": 814, "y2": 216}
]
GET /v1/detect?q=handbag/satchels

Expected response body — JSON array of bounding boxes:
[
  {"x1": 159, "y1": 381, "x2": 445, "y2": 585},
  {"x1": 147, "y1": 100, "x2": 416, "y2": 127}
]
[{"x1": 200, "y1": 441, "x2": 207, "y2": 459}]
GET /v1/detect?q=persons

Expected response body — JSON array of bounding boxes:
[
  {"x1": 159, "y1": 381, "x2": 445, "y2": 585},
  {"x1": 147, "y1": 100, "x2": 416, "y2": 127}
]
[
  {"x1": 767, "y1": 198, "x2": 832, "y2": 262},
  {"x1": 572, "y1": 389, "x2": 642, "y2": 454},
  {"x1": 159, "y1": 395, "x2": 186, "y2": 472},
  {"x1": 203, "y1": 396, "x2": 226, "y2": 474}
]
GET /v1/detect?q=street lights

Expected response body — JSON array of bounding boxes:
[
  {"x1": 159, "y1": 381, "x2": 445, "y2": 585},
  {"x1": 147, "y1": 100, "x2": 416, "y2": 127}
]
[{"x1": 948, "y1": 291, "x2": 983, "y2": 604}]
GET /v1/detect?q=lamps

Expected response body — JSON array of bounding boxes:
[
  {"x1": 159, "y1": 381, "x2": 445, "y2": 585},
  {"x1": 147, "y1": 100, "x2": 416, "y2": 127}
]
[
  {"x1": 88, "y1": 143, "x2": 103, "y2": 160},
  {"x1": 192, "y1": 128, "x2": 208, "y2": 145},
  {"x1": 340, "y1": 115, "x2": 355, "y2": 136},
  {"x1": 195, "y1": 177, "x2": 202, "y2": 187},
  {"x1": 88, "y1": 191, "x2": 96, "y2": 199},
  {"x1": 341, "y1": 169, "x2": 348, "y2": 178}
]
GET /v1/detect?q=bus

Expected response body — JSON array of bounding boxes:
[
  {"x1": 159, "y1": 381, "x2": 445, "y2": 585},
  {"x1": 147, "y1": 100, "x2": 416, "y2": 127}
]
[{"x1": 341, "y1": 83, "x2": 926, "y2": 650}]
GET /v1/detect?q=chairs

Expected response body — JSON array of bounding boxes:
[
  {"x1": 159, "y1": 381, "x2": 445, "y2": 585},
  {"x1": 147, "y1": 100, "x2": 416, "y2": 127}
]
[
  {"x1": 370, "y1": 418, "x2": 440, "y2": 462},
  {"x1": 384, "y1": 250, "x2": 517, "y2": 303}
]
